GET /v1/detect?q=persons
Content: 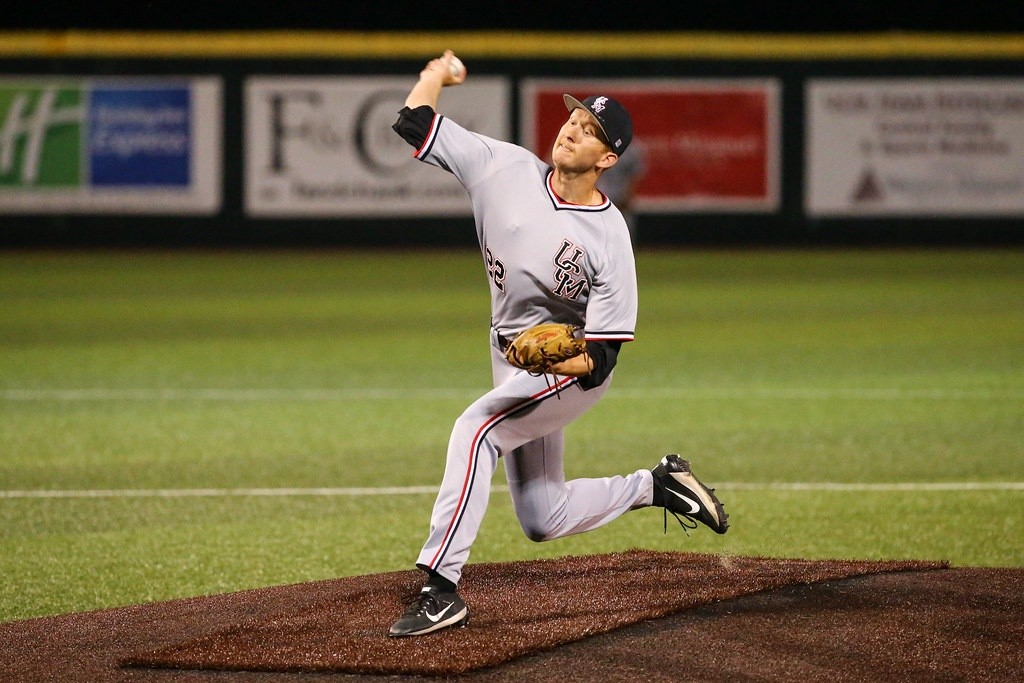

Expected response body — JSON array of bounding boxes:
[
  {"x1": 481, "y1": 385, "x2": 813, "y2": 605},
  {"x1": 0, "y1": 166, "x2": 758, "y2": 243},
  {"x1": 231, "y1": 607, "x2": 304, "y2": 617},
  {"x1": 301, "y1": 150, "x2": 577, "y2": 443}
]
[{"x1": 386, "y1": 49, "x2": 730, "y2": 635}]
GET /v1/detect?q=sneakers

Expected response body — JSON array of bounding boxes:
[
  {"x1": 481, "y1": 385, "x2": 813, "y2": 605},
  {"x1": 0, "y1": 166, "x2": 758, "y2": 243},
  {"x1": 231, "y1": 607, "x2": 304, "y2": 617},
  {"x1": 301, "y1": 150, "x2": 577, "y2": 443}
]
[
  {"x1": 389, "y1": 586, "x2": 468, "y2": 635},
  {"x1": 654, "y1": 454, "x2": 730, "y2": 534}
]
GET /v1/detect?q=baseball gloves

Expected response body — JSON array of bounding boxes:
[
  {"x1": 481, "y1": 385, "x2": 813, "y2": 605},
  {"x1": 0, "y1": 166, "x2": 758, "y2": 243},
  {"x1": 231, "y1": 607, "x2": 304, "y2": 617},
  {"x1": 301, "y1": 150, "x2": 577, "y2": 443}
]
[{"x1": 503, "y1": 322, "x2": 593, "y2": 400}]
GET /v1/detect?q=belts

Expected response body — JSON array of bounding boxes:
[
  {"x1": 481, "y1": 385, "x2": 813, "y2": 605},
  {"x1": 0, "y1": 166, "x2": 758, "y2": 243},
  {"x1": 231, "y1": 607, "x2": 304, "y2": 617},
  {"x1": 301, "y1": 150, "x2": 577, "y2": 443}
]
[{"x1": 496, "y1": 333, "x2": 513, "y2": 353}]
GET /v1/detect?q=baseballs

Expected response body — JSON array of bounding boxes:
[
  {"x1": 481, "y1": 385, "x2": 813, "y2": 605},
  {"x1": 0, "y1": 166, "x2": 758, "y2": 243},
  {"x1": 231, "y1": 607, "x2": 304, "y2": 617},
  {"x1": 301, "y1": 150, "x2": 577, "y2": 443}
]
[{"x1": 440, "y1": 55, "x2": 463, "y2": 76}]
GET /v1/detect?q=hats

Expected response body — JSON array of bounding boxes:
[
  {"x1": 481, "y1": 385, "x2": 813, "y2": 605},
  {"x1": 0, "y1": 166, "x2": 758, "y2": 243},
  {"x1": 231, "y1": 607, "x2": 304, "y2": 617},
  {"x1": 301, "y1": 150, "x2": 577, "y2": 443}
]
[{"x1": 563, "y1": 93, "x2": 632, "y2": 157}]
